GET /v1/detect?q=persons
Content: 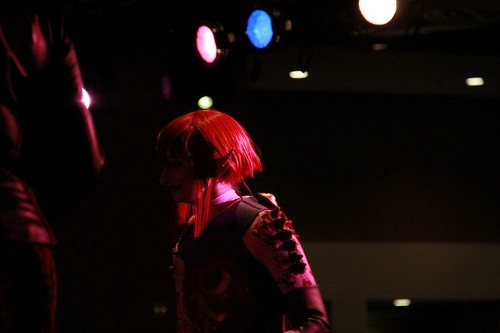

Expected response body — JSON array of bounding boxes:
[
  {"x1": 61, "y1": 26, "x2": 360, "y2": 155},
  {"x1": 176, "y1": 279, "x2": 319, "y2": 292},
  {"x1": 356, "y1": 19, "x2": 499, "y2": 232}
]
[{"x1": 148, "y1": 101, "x2": 330, "y2": 333}]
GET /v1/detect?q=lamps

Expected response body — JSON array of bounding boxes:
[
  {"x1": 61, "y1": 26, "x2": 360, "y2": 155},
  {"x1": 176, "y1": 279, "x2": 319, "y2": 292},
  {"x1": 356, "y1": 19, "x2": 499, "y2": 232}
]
[
  {"x1": 197, "y1": 20, "x2": 251, "y2": 65},
  {"x1": 246, "y1": 7, "x2": 307, "y2": 48},
  {"x1": 358, "y1": 0, "x2": 412, "y2": 29}
]
